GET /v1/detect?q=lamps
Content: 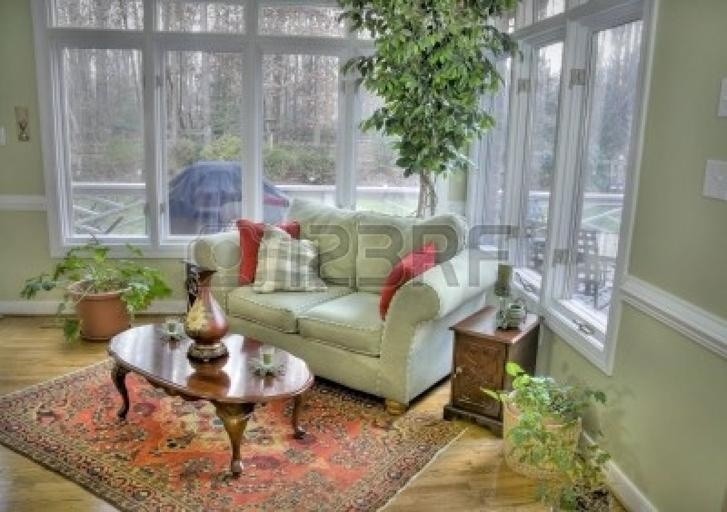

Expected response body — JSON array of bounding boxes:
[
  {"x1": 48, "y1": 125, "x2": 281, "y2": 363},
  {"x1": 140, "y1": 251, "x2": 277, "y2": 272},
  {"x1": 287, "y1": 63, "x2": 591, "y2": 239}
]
[
  {"x1": 493, "y1": 263, "x2": 513, "y2": 327},
  {"x1": 13, "y1": 106, "x2": 29, "y2": 141}
]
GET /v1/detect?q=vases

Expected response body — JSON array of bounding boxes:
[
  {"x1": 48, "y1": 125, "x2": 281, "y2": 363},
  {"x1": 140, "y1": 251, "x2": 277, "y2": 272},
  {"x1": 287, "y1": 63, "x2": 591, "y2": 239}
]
[{"x1": 183, "y1": 266, "x2": 230, "y2": 361}]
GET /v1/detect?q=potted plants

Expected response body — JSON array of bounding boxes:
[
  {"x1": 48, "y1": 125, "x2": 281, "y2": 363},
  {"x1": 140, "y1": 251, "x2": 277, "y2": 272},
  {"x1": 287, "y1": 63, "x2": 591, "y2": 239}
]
[
  {"x1": 20, "y1": 242, "x2": 172, "y2": 342},
  {"x1": 477, "y1": 360, "x2": 613, "y2": 512}
]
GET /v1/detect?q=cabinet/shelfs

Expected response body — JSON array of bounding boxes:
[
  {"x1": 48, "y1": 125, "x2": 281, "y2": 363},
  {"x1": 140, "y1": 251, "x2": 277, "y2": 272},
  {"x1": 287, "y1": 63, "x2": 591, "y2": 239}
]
[{"x1": 443, "y1": 305, "x2": 545, "y2": 438}]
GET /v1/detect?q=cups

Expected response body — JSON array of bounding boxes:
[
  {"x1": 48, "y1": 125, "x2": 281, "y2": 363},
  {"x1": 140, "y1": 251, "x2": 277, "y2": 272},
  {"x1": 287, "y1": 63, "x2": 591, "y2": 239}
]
[
  {"x1": 260, "y1": 345, "x2": 274, "y2": 367},
  {"x1": 261, "y1": 376, "x2": 276, "y2": 396},
  {"x1": 164, "y1": 315, "x2": 180, "y2": 333},
  {"x1": 166, "y1": 341, "x2": 180, "y2": 353}
]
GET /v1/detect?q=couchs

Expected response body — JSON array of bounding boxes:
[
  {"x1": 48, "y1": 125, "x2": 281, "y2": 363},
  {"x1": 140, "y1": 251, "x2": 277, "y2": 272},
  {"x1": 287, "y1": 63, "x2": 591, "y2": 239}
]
[{"x1": 193, "y1": 196, "x2": 508, "y2": 416}]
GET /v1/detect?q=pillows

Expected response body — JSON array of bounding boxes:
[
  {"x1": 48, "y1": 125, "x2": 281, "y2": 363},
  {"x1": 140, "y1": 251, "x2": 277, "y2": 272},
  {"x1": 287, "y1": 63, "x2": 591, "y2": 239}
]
[
  {"x1": 236, "y1": 219, "x2": 300, "y2": 284},
  {"x1": 252, "y1": 224, "x2": 329, "y2": 294},
  {"x1": 379, "y1": 239, "x2": 436, "y2": 321}
]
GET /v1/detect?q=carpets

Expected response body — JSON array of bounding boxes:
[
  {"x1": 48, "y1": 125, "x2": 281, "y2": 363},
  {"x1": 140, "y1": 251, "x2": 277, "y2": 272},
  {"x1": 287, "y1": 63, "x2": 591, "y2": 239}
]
[{"x1": 0, "y1": 358, "x2": 475, "y2": 512}]
